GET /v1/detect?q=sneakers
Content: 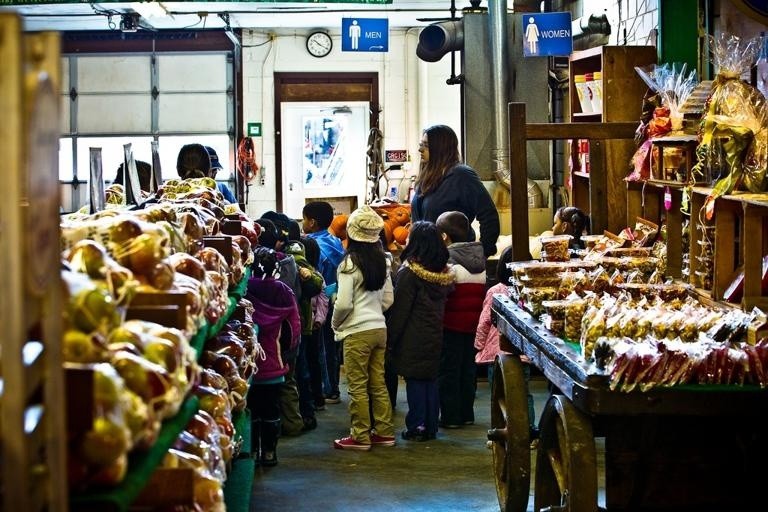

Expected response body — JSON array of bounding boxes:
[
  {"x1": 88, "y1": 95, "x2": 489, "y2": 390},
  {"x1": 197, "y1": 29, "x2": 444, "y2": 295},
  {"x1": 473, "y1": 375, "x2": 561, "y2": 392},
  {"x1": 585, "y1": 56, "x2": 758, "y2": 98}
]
[
  {"x1": 437, "y1": 419, "x2": 460, "y2": 429},
  {"x1": 365, "y1": 434, "x2": 396, "y2": 447},
  {"x1": 322, "y1": 393, "x2": 339, "y2": 404},
  {"x1": 250, "y1": 449, "x2": 261, "y2": 466},
  {"x1": 460, "y1": 418, "x2": 474, "y2": 425},
  {"x1": 402, "y1": 429, "x2": 426, "y2": 442},
  {"x1": 205, "y1": 146, "x2": 223, "y2": 170},
  {"x1": 261, "y1": 449, "x2": 277, "y2": 467},
  {"x1": 334, "y1": 436, "x2": 371, "y2": 450}
]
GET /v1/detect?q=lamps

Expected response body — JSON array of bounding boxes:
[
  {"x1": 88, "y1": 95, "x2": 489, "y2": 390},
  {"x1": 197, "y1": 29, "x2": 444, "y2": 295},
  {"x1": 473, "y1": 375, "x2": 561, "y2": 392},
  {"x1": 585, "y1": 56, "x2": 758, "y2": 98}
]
[{"x1": 372, "y1": 162, "x2": 411, "y2": 201}]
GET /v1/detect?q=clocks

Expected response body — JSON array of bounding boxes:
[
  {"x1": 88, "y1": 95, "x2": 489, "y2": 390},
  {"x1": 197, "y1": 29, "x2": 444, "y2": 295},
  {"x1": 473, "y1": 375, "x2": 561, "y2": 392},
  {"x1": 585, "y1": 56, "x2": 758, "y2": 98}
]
[{"x1": 306, "y1": 31, "x2": 332, "y2": 57}]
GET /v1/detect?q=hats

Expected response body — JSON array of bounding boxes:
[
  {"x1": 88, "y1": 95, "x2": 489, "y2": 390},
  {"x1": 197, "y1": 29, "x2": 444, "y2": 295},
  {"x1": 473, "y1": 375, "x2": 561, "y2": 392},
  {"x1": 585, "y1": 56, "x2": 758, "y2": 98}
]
[{"x1": 346, "y1": 206, "x2": 384, "y2": 242}]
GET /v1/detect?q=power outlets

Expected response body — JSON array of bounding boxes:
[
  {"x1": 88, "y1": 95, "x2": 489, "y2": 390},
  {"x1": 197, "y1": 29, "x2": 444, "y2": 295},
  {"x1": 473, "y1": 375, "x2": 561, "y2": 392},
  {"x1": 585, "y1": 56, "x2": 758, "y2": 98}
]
[{"x1": 261, "y1": 178, "x2": 265, "y2": 185}]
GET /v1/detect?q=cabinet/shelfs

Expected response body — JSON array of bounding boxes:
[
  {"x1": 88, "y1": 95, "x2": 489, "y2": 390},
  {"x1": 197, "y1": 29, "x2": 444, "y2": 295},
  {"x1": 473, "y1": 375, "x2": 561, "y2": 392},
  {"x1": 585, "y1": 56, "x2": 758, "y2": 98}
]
[
  {"x1": 566, "y1": 46, "x2": 656, "y2": 235},
  {"x1": 623, "y1": 130, "x2": 768, "y2": 326}
]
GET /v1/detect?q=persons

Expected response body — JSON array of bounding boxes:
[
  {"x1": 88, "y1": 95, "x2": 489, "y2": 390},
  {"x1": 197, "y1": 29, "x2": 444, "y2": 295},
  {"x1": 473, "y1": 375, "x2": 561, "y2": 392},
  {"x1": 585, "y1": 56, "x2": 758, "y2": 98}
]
[
  {"x1": 302, "y1": 201, "x2": 346, "y2": 404},
  {"x1": 203, "y1": 145, "x2": 238, "y2": 205},
  {"x1": 436, "y1": 211, "x2": 488, "y2": 430},
  {"x1": 410, "y1": 125, "x2": 501, "y2": 260},
  {"x1": 300, "y1": 237, "x2": 329, "y2": 411},
  {"x1": 386, "y1": 220, "x2": 457, "y2": 441},
  {"x1": 473, "y1": 244, "x2": 539, "y2": 450},
  {"x1": 274, "y1": 239, "x2": 325, "y2": 433},
  {"x1": 244, "y1": 246, "x2": 302, "y2": 472},
  {"x1": 261, "y1": 211, "x2": 301, "y2": 241},
  {"x1": 368, "y1": 227, "x2": 401, "y2": 430},
  {"x1": 175, "y1": 144, "x2": 211, "y2": 180},
  {"x1": 254, "y1": 219, "x2": 305, "y2": 437},
  {"x1": 551, "y1": 206, "x2": 591, "y2": 260},
  {"x1": 331, "y1": 205, "x2": 396, "y2": 451}
]
